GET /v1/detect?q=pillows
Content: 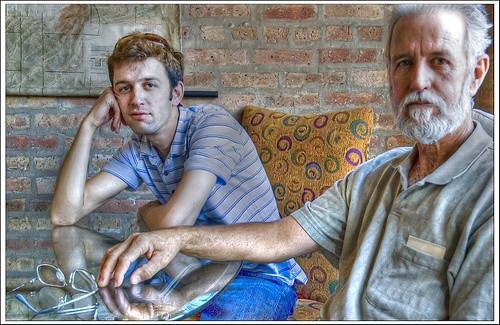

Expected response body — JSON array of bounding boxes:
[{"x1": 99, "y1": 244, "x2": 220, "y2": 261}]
[{"x1": 241, "y1": 105, "x2": 374, "y2": 303}]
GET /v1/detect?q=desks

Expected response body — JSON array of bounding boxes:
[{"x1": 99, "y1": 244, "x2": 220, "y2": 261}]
[{"x1": 6, "y1": 212, "x2": 241, "y2": 321}]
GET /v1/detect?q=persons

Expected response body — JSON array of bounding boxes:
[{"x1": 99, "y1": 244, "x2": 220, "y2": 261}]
[
  {"x1": 49, "y1": 31, "x2": 309, "y2": 321},
  {"x1": 52, "y1": 223, "x2": 243, "y2": 321},
  {"x1": 95, "y1": 3, "x2": 493, "y2": 320}
]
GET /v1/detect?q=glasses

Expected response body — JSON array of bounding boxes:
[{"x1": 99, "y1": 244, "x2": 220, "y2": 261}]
[
  {"x1": 6, "y1": 263, "x2": 100, "y2": 314},
  {"x1": 5, "y1": 287, "x2": 98, "y2": 320}
]
